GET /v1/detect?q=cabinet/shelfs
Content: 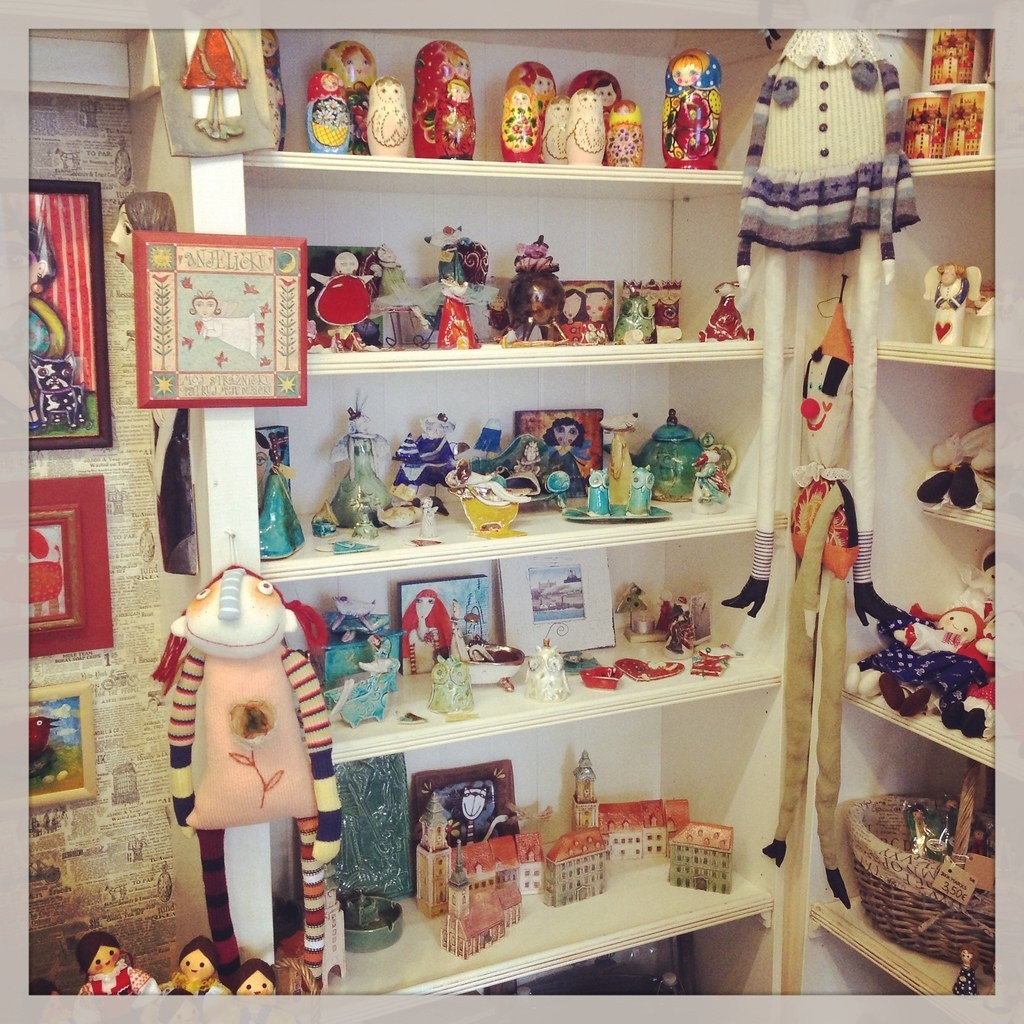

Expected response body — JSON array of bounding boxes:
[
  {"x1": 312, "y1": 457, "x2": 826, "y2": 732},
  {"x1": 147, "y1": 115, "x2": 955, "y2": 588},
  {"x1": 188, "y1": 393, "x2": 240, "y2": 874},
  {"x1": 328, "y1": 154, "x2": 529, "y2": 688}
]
[{"x1": 16, "y1": 25, "x2": 998, "y2": 1003}]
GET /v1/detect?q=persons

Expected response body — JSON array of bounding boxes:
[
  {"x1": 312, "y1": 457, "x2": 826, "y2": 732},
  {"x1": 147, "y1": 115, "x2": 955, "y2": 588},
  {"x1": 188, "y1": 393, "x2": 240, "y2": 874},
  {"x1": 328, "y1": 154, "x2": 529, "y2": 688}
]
[
  {"x1": 952, "y1": 944, "x2": 980, "y2": 995},
  {"x1": 767, "y1": 294, "x2": 866, "y2": 909},
  {"x1": 719, "y1": 29, "x2": 917, "y2": 626},
  {"x1": 30, "y1": 932, "x2": 319, "y2": 1024},
  {"x1": 150, "y1": 566, "x2": 343, "y2": 996},
  {"x1": 852, "y1": 395, "x2": 997, "y2": 741}
]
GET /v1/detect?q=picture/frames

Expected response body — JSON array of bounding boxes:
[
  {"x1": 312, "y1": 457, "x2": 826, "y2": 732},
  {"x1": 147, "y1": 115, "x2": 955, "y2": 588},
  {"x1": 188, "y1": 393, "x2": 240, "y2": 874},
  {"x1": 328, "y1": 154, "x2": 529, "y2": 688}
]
[
  {"x1": 501, "y1": 548, "x2": 614, "y2": 656},
  {"x1": 28, "y1": 474, "x2": 115, "y2": 658},
  {"x1": 28, "y1": 178, "x2": 116, "y2": 452},
  {"x1": 28, "y1": 679, "x2": 100, "y2": 807}
]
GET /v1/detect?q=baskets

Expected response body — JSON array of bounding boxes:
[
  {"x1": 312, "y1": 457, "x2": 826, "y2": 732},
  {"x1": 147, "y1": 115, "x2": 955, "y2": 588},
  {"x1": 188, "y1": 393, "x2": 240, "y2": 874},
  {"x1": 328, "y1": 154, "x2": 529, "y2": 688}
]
[{"x1": 844, "y1": 758, "x2": 995, "y2": 971}]
